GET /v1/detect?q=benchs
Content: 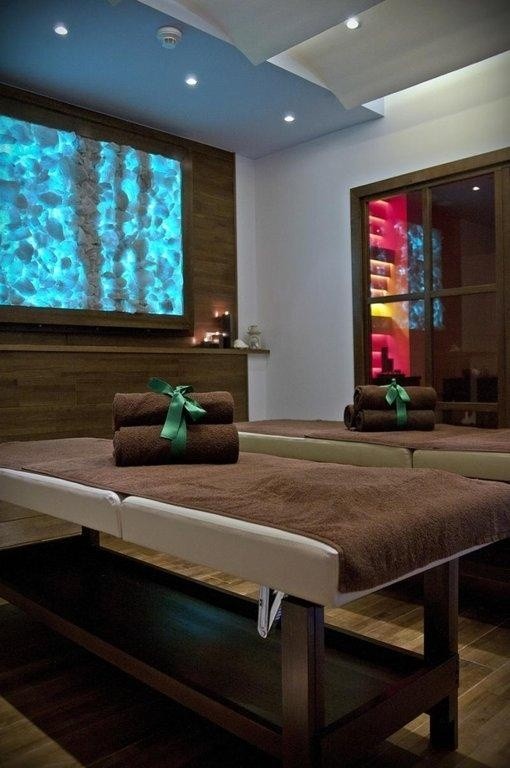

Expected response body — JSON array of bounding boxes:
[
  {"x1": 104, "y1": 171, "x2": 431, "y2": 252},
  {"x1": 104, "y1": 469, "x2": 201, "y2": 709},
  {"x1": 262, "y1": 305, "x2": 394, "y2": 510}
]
[
  {"x1": 232, "y1": 419, "x2": 508, "y2": 484},
  {"x1": 0, "y1": 438, "x2": 508, "y2": 767}
]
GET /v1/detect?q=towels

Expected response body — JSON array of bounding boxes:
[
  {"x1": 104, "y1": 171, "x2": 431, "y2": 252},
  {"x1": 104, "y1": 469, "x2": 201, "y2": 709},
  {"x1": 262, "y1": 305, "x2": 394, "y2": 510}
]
[
  {"x1": 113, "y1": 390, "x2": 239, "y2": 465},
  {"x1": 344, "y1": 384, "x2": 437, "y2": 432}
]
